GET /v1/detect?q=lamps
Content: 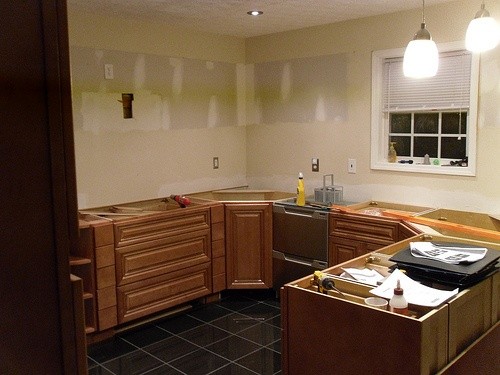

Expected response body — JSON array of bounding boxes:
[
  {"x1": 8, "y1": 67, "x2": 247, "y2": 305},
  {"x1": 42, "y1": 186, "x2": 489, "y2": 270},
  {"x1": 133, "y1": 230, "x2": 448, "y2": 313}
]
[
  {"x1": 403, "y1": 0, "x2": 439, "y2": 78},
  {"x1": 465, "y1": 0, "x2": 500, "y2": 54}
]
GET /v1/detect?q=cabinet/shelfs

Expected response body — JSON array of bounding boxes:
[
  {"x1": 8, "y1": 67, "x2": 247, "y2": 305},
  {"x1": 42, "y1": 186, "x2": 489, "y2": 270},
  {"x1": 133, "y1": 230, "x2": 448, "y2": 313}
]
[{"x1": 70, "y1": 190, "x2": 500, "y2": 375}]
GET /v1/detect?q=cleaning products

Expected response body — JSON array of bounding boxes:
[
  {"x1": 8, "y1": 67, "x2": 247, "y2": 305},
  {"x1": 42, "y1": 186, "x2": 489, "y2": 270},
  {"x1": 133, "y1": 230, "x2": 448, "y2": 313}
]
[{"x1": 296, "y1": 172, "x2": 305, "y2": 206}]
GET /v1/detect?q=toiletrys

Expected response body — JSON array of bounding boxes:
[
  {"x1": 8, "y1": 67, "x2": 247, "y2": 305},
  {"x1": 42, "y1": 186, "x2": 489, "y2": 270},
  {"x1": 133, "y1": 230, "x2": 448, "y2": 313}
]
[{"x1": 387, "y1": 142, "x2": 397, "y2": 162}]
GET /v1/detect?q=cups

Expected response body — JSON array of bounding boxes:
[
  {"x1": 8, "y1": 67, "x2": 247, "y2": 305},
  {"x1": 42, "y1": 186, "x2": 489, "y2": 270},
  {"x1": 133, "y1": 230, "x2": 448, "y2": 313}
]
[{"x1": 364, "y1": 297, "x2": 388, "y2": 310}]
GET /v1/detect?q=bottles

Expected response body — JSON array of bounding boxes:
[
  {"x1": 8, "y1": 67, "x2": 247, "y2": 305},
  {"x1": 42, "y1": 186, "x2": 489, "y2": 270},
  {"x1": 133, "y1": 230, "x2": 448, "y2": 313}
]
[
  {"x1": 170, "y1": 195, "x2": 190, "y2": 205},
  {"x1": 389, "y1": 279, "x2": 408, "y2": 316},
  {"x1": 296, "y1": 172, "x2": 305, "y2": 206},
  {"x1": 424, "y1": 154, "x2": 429, "y2": 164}
]
